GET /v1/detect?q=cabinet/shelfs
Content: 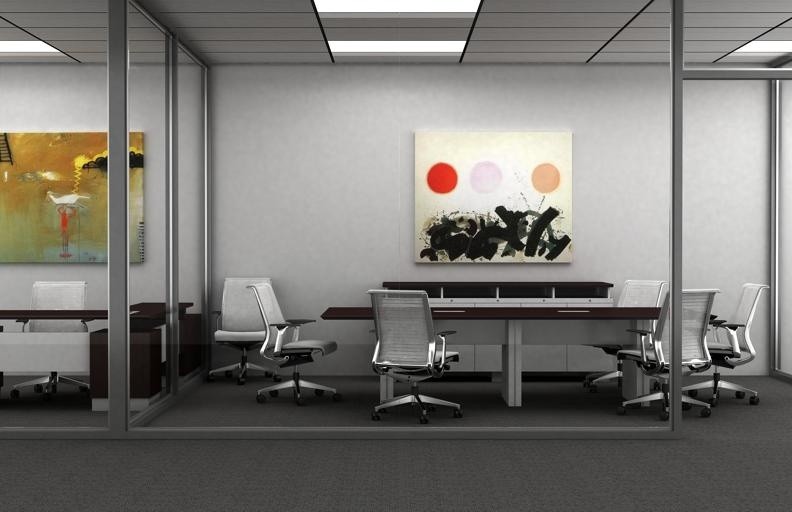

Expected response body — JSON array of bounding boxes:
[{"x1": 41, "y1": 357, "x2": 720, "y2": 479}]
[{"x1": 87, "y1": 330, "x2": 163, "y2": 414}]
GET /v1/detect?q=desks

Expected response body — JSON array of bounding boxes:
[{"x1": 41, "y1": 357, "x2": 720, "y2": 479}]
[
  {"x1": 1, "y1": 300, "x2": 197, "y2": 404},
  {"x1": 319, "y1": 305, "x2": 664, "y2": 409}
]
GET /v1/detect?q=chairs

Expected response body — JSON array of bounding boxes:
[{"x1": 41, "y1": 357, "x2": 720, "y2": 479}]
[
  {"x1": 581, "y1": 280, "x2": 670, "y2": 398},
  {"x1": 613, "y1": 283, "x2": 721, "y2": 423},
  {"x1": 367, "y1": 290, "x2": 464, "y2": 424},
  {"x1": 684, "y1": 283, "x2": 769, "y2": 406},
  {"x1": 205, "y1": 278, "x2": 278, "y2": 388},
  {"x1": 248, "y1": 283, "x2": 343, "y2": 405},
  {"x1": 9, "y1": 281, "x2": 90, "y2": 402}
]
